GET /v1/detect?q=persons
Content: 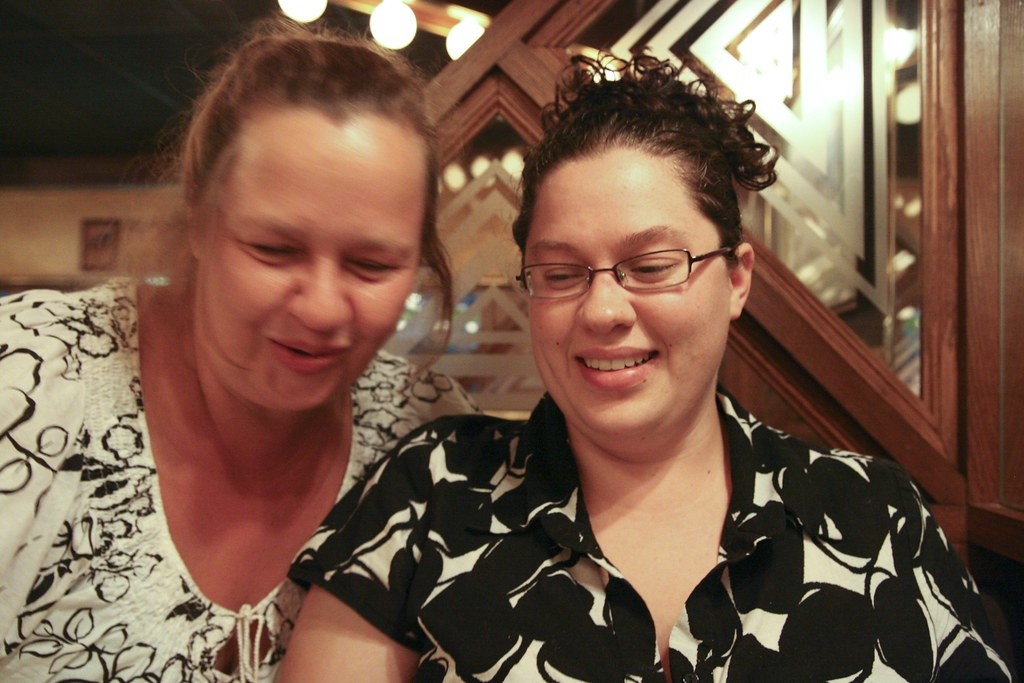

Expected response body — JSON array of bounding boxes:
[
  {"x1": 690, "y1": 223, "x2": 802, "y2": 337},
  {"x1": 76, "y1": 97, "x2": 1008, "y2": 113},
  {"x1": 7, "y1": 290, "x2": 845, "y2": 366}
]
[
  {"x1": 274, "y1": 45, "x2": 1024, "y2": 683},
  {"x1": 1, "y1": 22, "x2": 481, "y2": 683}
]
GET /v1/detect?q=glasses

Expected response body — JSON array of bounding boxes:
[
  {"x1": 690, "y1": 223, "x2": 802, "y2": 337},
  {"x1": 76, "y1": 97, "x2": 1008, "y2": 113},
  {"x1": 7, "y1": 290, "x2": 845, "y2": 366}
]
[{"x1": 515, "y1": 246, "x2": 731, "y2": 301}]
